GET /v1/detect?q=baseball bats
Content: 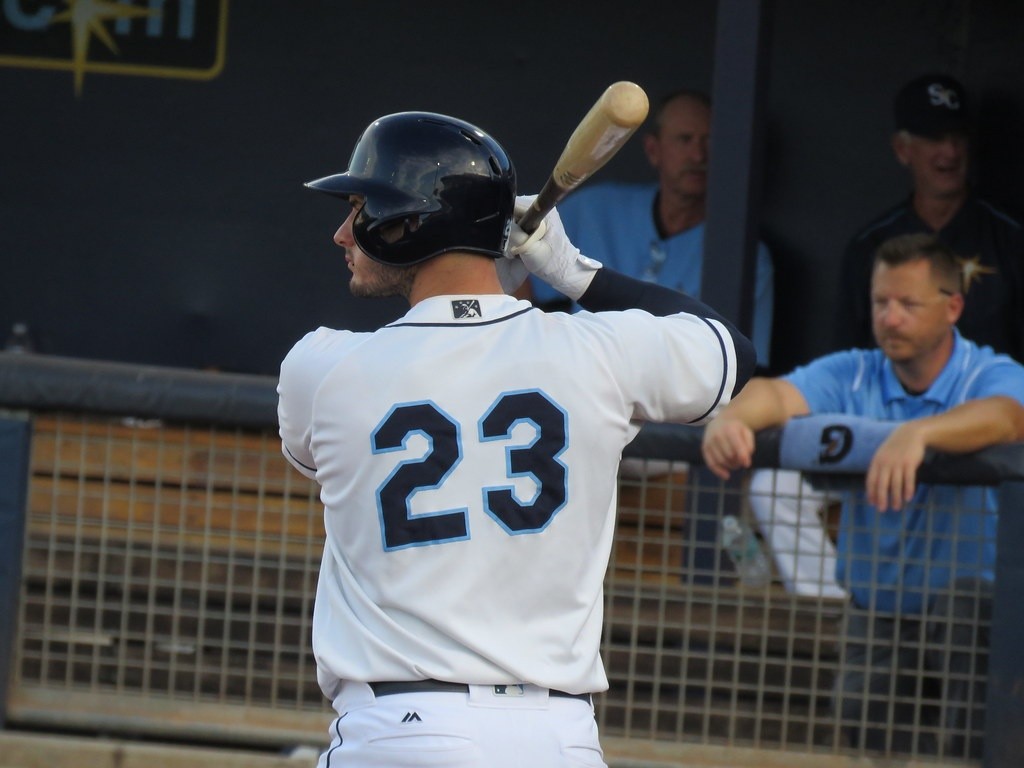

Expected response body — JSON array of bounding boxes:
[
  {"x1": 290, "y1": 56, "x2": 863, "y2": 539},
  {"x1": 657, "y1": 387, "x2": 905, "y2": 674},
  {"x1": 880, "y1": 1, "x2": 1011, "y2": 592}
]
[{"x1": 504, "y1": 79, "x2": 650, "y2": 261}]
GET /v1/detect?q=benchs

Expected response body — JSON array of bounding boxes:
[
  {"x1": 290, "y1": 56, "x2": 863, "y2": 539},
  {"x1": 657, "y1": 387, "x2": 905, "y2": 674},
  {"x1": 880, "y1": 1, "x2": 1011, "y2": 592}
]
[{"x1": 23, "y1": 419, "x2": 842, "y2": 715}]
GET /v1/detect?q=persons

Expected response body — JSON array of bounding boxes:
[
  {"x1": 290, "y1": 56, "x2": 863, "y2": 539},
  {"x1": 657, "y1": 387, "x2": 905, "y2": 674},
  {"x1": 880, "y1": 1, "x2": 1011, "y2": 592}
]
[
  {"x1": 702, "y1": 234, "x2": 1024, "y2": 759},
  {"x1": 512, "y1": 90, "x2": 773, "y2": 584},
  {"x1": 834, "y1": 77, "x2": 1023, "y2": 361},
  {"x1": 275, "y1": 112, "x2": 756, "y2": 768}
]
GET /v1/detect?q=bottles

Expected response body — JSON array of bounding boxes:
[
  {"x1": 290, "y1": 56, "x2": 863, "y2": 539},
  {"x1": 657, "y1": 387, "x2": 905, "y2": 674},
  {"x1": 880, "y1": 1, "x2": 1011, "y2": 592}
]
[{"x1": 724, "y1": 516, "x2": 767, "y2": 587}]
